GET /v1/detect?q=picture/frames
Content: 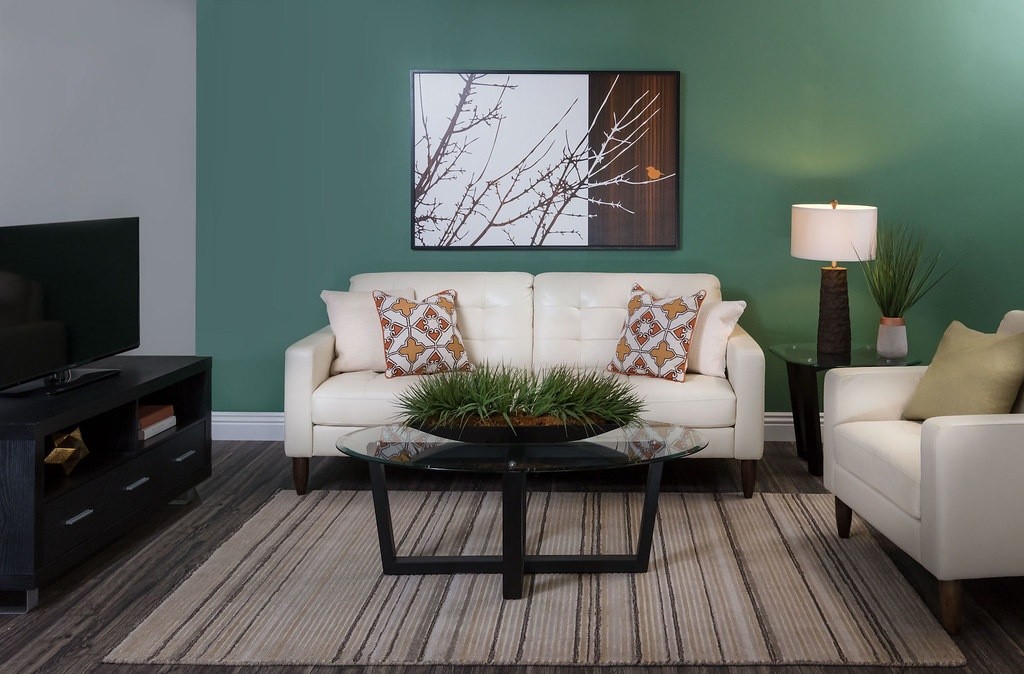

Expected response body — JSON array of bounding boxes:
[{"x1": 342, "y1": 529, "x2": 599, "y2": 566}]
[{"x1": 409, "y1": 70, "x2": 681, "y2": 249}]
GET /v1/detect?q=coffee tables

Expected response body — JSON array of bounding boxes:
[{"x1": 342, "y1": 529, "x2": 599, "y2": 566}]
[{"x1": 333, "y1": 420, "x2": 709, "y2": 598}]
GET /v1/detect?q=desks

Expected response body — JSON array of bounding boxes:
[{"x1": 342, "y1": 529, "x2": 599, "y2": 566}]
[{"x1": 771, "y1": 342, "x2": 922, "y2": 476}]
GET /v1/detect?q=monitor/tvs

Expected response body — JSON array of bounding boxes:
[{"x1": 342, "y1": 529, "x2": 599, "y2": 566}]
[{"x1": 0, "y1": 217, "x2": 141, "y2": 397}]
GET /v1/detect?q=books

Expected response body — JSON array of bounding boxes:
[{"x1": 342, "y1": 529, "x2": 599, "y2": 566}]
[
  {"x1": 138, "y1": 415, "x2": 177, "y2": 440},
  {"x1": 137, "y1": 404, "x2": 174, "y2": 429}
]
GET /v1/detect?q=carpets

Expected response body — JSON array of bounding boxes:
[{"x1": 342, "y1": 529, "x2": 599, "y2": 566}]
[{"x1": 102, "y1": 488, "x2": 967, "y2": 667}]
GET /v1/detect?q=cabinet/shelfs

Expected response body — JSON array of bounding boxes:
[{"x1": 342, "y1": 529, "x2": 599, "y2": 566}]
[{"x1": 0, "y1": 355, "x2": 213, "y2": 619}]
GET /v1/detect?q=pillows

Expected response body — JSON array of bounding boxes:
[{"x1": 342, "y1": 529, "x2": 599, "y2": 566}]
[
  {"x1": 372, "y1": 291, "x2": 477, "y2": 379},
  {"x1": 607, "y1": 283, "x2": 706, "y2": 382},
  {"x1": 899, "y1": 322, "x2": 1023, "y2": 421},
  {"x1": 680, "y1": 299, "x2": 747, "y2": 378},
  {"x1": 320, "y1": 290, "x2": 416, "y2": 374}
]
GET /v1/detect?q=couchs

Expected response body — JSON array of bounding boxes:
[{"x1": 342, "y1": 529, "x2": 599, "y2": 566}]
[
  {"x1": 822, "y1": 309, "x2": 1023, "y2": 632},
  {"x1": 285, "y1": 271, "x2": 765, "y2": 498}
]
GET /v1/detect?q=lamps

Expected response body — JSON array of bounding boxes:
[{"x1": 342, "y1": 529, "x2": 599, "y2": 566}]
[{"x1": 791, "y1": 199, "x2": 877, "y2": 356}]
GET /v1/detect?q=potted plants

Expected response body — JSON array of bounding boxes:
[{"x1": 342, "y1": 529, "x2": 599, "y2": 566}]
[
  {"x1": 845, "y1": 219, "x2": 961, "y2": 360},
  {"x1": 389, "y1": 366, "x2": 652, "y2": 444}
]
